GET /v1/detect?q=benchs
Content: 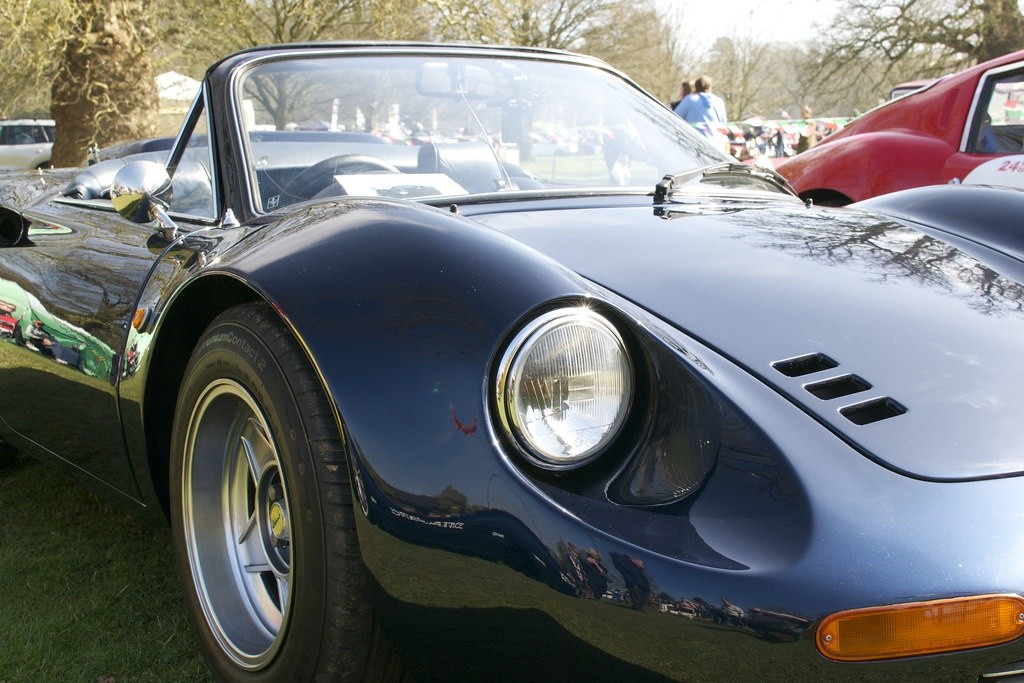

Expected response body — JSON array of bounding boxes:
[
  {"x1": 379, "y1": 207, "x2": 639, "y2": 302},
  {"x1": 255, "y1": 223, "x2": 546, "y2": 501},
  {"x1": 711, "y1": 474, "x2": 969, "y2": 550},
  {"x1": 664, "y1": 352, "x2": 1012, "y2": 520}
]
[{"x1": 74, "y1": 141, "x2": 418, "y2": 216}]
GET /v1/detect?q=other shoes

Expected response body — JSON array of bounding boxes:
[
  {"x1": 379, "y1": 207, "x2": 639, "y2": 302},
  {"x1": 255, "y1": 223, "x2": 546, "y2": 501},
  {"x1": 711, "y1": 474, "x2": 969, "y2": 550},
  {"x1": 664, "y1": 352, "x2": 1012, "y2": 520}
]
[{"x1": 56, "y1": 358, "x2": 67, "y2": 364}]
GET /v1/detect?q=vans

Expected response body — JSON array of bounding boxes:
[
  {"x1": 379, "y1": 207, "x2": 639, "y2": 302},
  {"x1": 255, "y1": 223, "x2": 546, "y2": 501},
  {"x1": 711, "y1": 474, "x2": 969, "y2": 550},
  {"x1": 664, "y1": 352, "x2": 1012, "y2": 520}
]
[{"x1": 0, "y1": 117, "x2": 64, "y2": 168}]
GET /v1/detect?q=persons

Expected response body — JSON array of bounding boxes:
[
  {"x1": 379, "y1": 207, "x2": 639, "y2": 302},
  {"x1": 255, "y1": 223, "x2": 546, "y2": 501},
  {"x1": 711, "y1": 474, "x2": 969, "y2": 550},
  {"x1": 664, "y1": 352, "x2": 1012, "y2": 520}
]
[
  {"x1": 412, "y1": 124, "x2": 469, "y2": 144},
  {"x1": 555, "y1": 543, "x2": 745, "y2": 626},
  {"x1": 728, "y1": 104, "x2": 817, "y2": 158},
  {"x1": 0, "y1": 300, "x2": 138, "y2": 375},
  {"x1": 673, "y1": 77, "x2": 726, "y2": 121},
  {"x1": 671, "y1": 80, "x2": 696, "y2": 109},
  {"x1": 976, "y1": 111, "x2": 998, "y2": 153}
]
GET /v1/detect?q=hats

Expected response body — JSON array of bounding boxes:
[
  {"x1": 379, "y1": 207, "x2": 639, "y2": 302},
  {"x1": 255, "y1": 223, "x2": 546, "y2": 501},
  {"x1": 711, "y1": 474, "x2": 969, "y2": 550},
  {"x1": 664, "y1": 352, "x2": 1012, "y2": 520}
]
[{"x1": 36, "y1": 321, "x2": 44, "y2": 325}]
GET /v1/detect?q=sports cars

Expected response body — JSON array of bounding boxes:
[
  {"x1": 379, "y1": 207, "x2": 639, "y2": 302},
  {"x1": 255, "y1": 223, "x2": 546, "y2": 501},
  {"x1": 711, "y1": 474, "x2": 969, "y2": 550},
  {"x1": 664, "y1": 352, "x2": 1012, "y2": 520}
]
[
  {"x1": 0, "y1": 39, "x2": 1023, "y2": 682},
  {"x1": 769, "y1": 47, "x2": 1022, "y2": 209}
]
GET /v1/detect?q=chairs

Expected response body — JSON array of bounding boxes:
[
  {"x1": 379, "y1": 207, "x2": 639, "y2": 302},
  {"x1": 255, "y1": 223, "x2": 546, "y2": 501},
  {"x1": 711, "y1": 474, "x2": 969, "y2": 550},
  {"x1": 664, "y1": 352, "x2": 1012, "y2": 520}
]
[
  {"x1": 417, "y1": 139, "x2": 504, "y2": 195},
  {"x1": 160, "y1": 161, "x2": 215, "y2": 219}
]
